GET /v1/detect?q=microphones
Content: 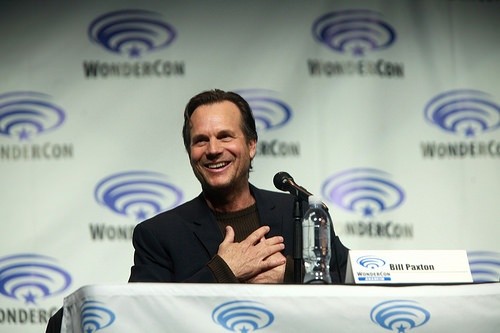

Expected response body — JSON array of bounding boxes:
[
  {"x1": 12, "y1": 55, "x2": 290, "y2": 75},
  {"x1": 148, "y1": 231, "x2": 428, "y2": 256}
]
[{"x1": 273, "y1": 171, "x2": 328, "y2": 213}]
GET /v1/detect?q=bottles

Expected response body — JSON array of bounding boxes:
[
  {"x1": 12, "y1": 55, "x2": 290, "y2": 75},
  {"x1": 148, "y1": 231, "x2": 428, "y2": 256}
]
[{"x1": 302, "y1": 194, "x2": 333, "y2": 287}]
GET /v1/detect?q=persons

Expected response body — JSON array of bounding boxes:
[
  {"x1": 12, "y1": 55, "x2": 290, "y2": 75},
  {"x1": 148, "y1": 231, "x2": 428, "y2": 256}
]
[{"x1": 128, "y1": 88, "x2": 352, "y2": 283}]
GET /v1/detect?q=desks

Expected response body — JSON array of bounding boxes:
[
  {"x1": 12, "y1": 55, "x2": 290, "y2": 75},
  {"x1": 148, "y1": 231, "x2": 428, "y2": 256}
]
[{"x1": 46, "y1": 281, "x2": 500, "y2": 332}]
